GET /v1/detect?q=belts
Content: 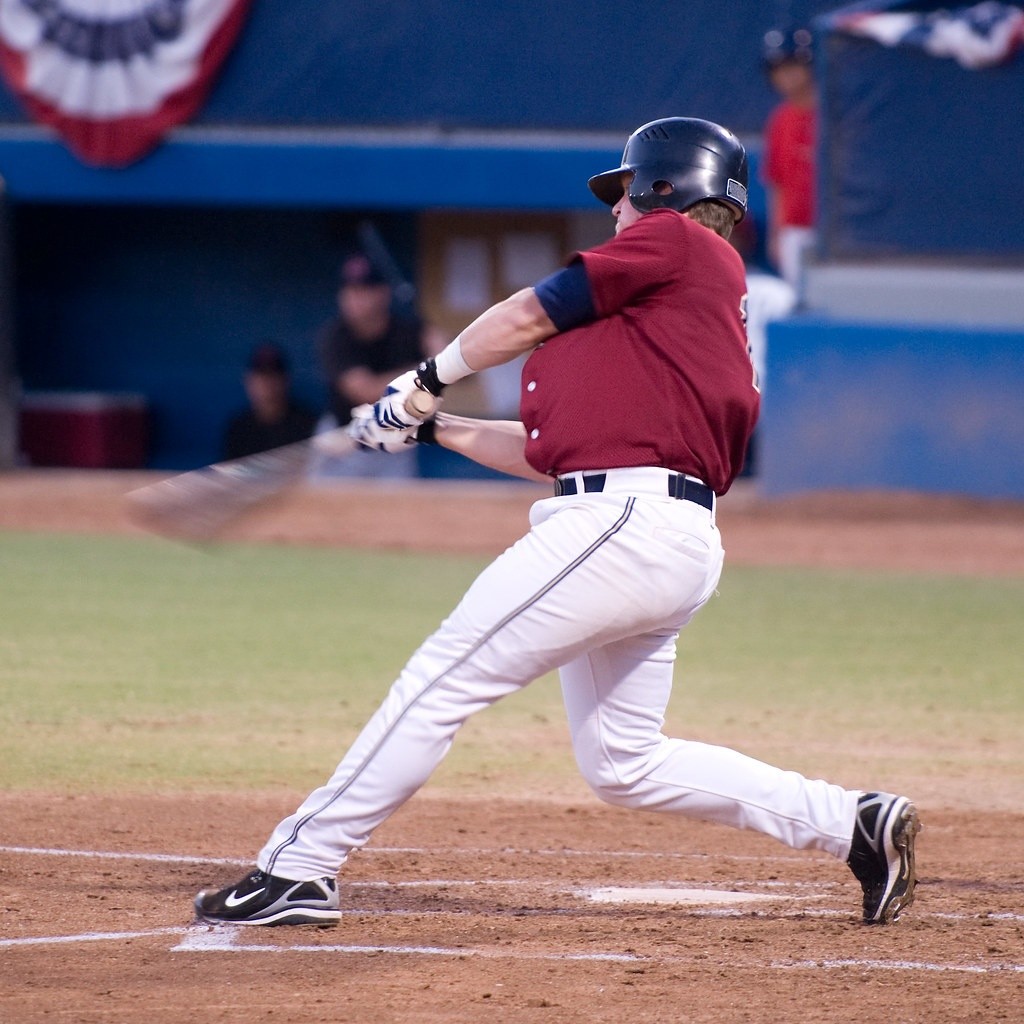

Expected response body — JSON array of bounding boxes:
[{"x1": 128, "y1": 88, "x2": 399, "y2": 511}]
[{"x1": 553, "y1": 474, "x2": 716, "y2": 510}]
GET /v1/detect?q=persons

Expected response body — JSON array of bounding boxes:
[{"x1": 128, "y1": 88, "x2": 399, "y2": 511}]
[
  {"x1": 215, "y1": 246, "x2": 435, "y2": 480},
  {"x1": 192, "y1": 117, "x2": 923, "y2": 927},
  {"x1": 761, "y1": 48, "x2": 815, "y2": 312}
]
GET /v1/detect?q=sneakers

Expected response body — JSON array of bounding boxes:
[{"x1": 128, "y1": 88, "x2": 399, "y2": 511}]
[
  {"x1": 196, "y1": 867, "x2": 345, "y2": 930},
  {"x1": 845, "y1": 789, "x2": 923, "y2": 924}
]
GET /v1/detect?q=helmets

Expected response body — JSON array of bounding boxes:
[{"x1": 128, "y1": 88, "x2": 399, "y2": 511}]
[
  {"x1": 753, "y1": 24, "x2": 819, "y2": 69},
  {"x1": 581, "y1": 116, "x2": 749, "y2": 223}
]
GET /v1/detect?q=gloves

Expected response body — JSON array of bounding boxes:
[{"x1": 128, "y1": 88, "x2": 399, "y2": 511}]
[
  {"x1": 374, "y1": 360, "x2": 448, "y2": 429},
  {"x1": 347, "y1": 400, "x2": 437, "y2": 456}
]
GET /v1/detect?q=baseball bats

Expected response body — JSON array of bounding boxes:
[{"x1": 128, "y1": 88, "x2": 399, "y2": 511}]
[{"x1": 123, "y1": 379, "x2": 438, "y2": 549}]
